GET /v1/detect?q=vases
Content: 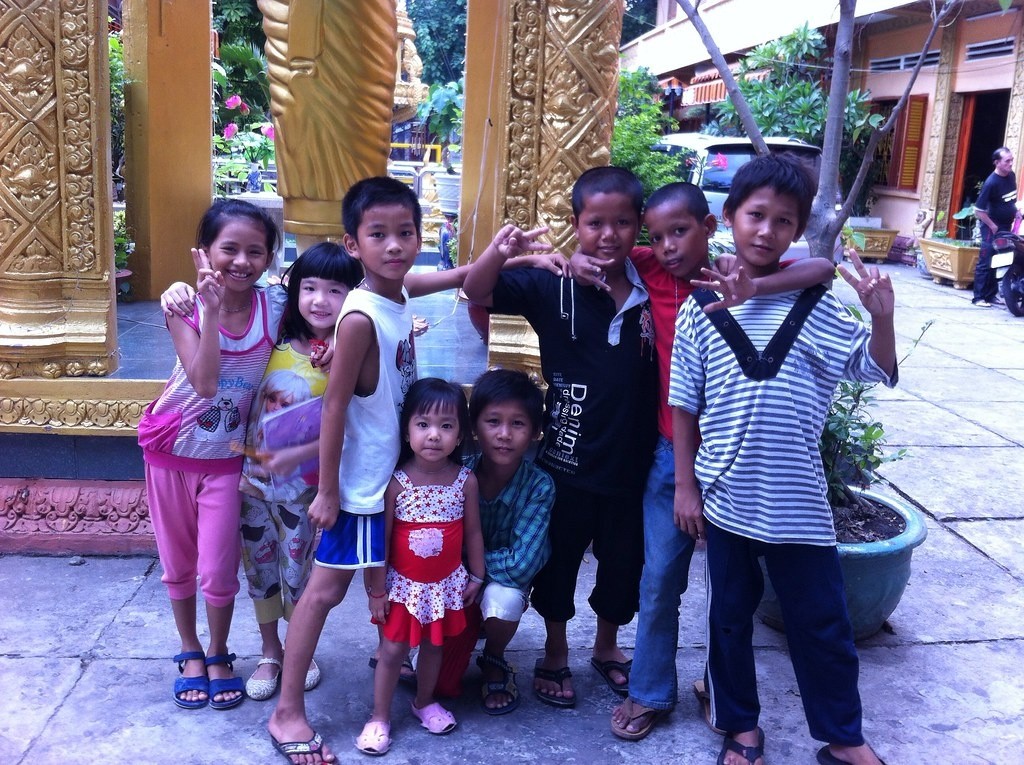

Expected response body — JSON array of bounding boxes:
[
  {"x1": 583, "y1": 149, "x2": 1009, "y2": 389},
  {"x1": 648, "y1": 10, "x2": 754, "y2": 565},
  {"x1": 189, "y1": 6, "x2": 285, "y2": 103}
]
[
  {"x1": 115, "y1": 269, "x2": 132, "y2": 294},
  {"x1": 247, "y1": 163, "x2": 262, "y2": 193},
  {"x1": 915, "y1": 249, "x2": 934, "y2": 279},
  {"x1": 436, "y1": 213, "x2": 457, "y2": 271}
]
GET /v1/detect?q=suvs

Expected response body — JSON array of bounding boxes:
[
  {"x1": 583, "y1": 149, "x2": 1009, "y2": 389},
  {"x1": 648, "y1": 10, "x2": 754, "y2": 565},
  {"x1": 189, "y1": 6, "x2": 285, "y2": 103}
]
[{"x1": 657, "y1": 131, "x2": 847, "y2": 265}]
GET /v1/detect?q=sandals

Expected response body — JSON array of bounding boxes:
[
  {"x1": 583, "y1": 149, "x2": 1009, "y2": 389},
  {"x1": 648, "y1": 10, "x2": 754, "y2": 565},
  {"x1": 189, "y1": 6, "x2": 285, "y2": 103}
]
[
  {"x1": 172, "y1": 648, "x2": 211, "y2": 710},
  {"x1": 476, "y1": 654, "x2": 520, "y2": 716},
  {"x1": 206, "y1": 652, "x2": 245, "y2": 709}
]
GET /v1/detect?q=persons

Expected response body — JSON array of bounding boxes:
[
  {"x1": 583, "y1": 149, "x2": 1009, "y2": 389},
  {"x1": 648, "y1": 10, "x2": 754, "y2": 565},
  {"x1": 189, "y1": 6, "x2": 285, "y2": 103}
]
[
  {"x1": 464, "y1": 164, "x2": 737, "y2": 704},
  {"x1": 567, "y1": 181, "x2": 835, "y2": 740},
  {"x1": 668, "y1": 153, "x2": 899, "y2": 765},
  {"x1": 136, "y1": 175, "x2": 556, "y2": 765},
  {"x1": 971, "y1": 147, "x2": 1017, "y2": 307}
]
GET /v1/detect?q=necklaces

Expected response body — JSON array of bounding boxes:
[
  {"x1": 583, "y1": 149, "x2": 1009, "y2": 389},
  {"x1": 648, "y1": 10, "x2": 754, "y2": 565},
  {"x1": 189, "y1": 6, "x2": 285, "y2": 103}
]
[
  {"x1": 361, "y1": 280, "x2": 374, "y2": 292},
  {"x1": 219, "y1": 304, "x2": 252, "y2": 312},
  {"x1": 410, "y1": 461, "x2": 452, "y2": 475}
]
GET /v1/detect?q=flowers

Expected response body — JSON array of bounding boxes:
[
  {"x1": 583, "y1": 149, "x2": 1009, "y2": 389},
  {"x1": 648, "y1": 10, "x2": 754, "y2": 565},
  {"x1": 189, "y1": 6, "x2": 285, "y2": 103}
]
[
  {"x1": 113, "y1": 214, "x2": 137, "y2": 274},
  {"x1": 223, "y1": 95, "x2": 276, "y2": 171},
  {"x1": 685, "y1": 152, "x2": 729, "y2": 188}
]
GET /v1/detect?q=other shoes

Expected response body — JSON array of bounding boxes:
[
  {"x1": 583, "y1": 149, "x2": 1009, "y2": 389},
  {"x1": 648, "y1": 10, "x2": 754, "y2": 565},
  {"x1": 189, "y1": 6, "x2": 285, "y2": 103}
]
[
  {"x1": 245, "y1": 648, "x2": 285, "y2": 701},
  {"x1": 304, "y1": 658, "x2": 321, "y2": 691},
  {"x1": 989, "y1": 297, "x2": 1005, "y2": 305},
  {"x1": 976, "y1": 300, "x2": 991, "y2": 307}
]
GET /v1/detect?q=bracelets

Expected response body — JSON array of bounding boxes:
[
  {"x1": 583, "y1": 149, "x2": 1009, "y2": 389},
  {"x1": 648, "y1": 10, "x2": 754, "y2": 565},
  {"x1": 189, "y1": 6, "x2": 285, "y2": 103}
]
[
  {"x1": 469, "y1": 572, "x2": 485, "y2": 584},
  {"x1": 368, "y1": 590, "x2": 387, "y2": 598}
]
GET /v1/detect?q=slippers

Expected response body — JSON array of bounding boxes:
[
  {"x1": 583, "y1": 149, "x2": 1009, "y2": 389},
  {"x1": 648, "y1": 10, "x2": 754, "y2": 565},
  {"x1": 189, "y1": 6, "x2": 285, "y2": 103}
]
[
  {"x1": 817, "y1": 744, "x2": 886, "y2": 765},
  {"x1": 533, "y1": 657, "x2": 577, "y2": 706},
  {"x1": 590, "y1": 656, "x2": 633, "y2": 692},
  {"x1": 717, "y1": 726, "x2": 764, "y2": 765},
  {"x1": 410, "y1": 696, "x2": 458, "y2": 736},
  {"x1": 610, "y1": 697, "x2": 675, "y2": 739},
  {"x1": 355, "y1": 719, "x2": 394, "y2": 756},
  {"x1": 367, "y1": 654, "x2": 418, "y2": 685},
  {"x1": 271, "y1": 731, "x2": 341, "y2": 765},
  {"x1": 693, "y1": 679, "x2": 728, "y2": 735}
]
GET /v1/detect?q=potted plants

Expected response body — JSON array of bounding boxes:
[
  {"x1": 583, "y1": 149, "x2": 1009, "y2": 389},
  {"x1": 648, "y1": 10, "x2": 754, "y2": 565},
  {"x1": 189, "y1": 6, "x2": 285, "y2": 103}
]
[
  {"x1": 753, "y1": 300, "x2": 943, "y2": 642},
  {"x1": 416, "y1": 77, "x2": 466, "y2": 212},
  {"x1": 920, "y1": 207, "x2": 984, "y2": 289}
]
